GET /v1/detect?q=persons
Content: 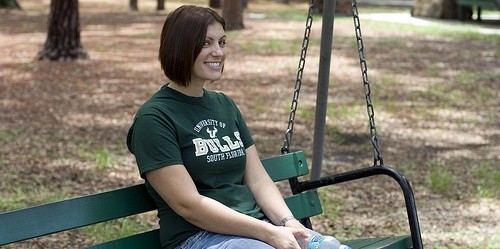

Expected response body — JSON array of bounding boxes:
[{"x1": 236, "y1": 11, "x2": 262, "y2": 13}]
[{"x1": 126, "y1": 5, "x2": 352, "y2": 249}]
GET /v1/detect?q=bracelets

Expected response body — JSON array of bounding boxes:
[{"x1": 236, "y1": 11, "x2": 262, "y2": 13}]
[{"x1": 277, "y1": 215, "x2": 298, "y2": 226}]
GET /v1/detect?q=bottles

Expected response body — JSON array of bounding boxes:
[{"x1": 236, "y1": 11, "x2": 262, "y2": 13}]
[{"x1": 296, "y1": 235, "x2": 352, "y2": 249}]
